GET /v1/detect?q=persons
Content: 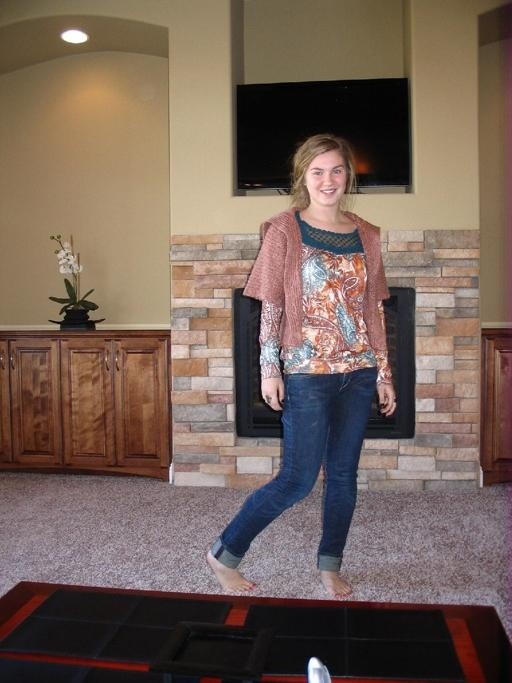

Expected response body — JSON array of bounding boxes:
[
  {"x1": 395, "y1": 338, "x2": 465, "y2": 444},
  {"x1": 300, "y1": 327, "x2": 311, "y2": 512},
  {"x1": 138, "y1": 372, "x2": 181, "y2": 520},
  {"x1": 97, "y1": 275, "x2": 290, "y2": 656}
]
[{"x1": 201, "y1": 131, "x2": 400, "y2": 598}]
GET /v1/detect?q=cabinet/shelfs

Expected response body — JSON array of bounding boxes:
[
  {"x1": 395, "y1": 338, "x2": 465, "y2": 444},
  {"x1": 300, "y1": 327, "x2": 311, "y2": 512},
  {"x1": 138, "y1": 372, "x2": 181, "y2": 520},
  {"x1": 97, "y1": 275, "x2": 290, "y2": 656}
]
[
  {"x1": 0, "y1": 336, "x2": 172, "y2": 481},
  {"x1": 480, "y1": 326, "x2": 512, "y2": 487}
]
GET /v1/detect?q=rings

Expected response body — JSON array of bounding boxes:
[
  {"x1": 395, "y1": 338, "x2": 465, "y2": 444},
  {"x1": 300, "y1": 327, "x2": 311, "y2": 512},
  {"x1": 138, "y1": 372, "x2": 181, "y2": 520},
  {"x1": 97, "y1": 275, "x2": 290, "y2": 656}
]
[
  {"x1": 393, "y1": 399, "x2": 397, "y2": 402},
  {"x1": 266, "y1": 395, "x2": 272, "y2": 403}
]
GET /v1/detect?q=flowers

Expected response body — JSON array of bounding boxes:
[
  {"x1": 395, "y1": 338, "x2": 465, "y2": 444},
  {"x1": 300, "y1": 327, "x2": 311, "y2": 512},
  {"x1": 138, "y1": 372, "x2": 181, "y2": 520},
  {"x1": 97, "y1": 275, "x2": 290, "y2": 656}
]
[{"x1": 47, "y1": 233, "x2": 100, "y2": 314}]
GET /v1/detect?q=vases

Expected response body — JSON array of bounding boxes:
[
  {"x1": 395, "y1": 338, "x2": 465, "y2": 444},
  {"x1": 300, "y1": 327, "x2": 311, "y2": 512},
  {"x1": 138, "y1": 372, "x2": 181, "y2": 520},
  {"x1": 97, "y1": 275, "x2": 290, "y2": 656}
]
[{"x1": 47, "y1": 310, "x2": 106, "y2": 331}]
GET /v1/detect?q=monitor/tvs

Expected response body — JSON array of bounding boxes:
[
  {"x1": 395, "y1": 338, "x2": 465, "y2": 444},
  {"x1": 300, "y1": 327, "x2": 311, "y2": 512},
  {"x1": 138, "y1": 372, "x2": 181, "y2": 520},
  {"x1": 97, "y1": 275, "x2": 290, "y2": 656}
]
[{"x1": 236, "y1": 76, "x2": 410, "y2": 189}]
